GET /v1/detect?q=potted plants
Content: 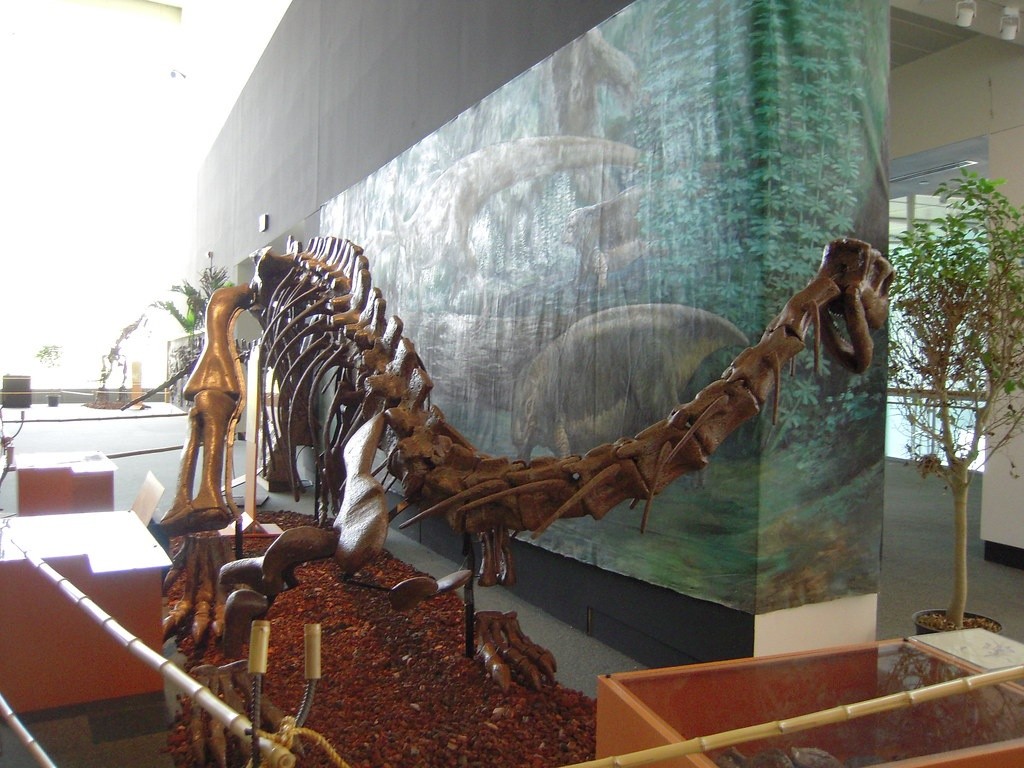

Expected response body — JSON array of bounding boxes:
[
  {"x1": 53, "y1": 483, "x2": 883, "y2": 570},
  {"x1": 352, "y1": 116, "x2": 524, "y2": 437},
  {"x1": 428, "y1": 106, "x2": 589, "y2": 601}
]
[{"x1": 875, "y1": 164, "x2": 1023, "y2": 636}]
